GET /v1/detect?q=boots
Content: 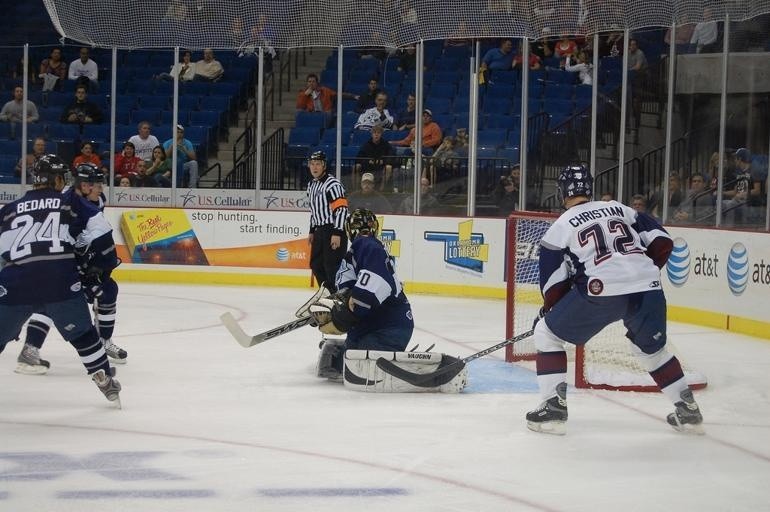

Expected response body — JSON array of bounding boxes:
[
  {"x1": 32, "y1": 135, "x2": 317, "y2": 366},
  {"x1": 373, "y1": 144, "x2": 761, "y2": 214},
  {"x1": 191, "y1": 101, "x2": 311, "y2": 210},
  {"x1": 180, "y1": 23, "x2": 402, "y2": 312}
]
[
  {"x1": 525, "y1": 381, "x2": 568, "y2": 423},
  {"x1": 99, "y1": 337, "x2": 127, "y2": 359},
  {"x1": 666, "y1": 388, "x2": 703, "y2": 427},
  {"x1": 92, "y1": 369, "x2": 122, "y2": 401},
  {"x1": 17, "y1": 342, "x2": 50, "y2": 368}
]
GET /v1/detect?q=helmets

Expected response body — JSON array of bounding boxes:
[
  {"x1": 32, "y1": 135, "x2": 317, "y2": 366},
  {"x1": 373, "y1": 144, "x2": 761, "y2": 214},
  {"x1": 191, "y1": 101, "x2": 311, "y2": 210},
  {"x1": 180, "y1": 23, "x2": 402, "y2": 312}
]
[
  {"x1": 30, "y1": 153, "x2": 71, "y2": 186},
  {"x1": 73, "y1": 162, "x2": 106, "y2": 190},
  {"x1": 554, "y1": 164, "x2": 594, "y2": 208},
  {"x1": 344, "y1": 208, "x2": 380, "y2": 243}
]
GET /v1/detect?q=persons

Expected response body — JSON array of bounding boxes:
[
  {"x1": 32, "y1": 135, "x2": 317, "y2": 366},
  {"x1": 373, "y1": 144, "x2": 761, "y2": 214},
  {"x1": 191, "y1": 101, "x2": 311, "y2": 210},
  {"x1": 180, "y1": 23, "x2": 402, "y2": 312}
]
[
  {"x1": 525, "y1": 166, "x2": 702, "y2": 426},
  {"x1": 17, "y1": 161, "x2": 127, "y2": 368},
  {"x1": 0, "y1": 154, "x2": 121, "y2": 401},
  {"x1": 309, "y1": 208, "x2": 413, "y2": 382},
  {"x1": 306, "y1": 150, "x2": 349, "y2": 294}
]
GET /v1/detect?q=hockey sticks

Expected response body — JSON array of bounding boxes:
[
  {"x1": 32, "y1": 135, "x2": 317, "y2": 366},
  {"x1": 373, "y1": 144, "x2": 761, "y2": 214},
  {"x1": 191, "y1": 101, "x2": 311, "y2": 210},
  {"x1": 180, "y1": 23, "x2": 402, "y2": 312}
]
[
  {"x1": 93, "y1": 296, "x2": 115, "y2": 377},
  {"x1": 376, "y1": 329, "x2": 534, "y2": 387},
  {"x1": 218, "y1": 312, "x2": 315, "y2": 348}
]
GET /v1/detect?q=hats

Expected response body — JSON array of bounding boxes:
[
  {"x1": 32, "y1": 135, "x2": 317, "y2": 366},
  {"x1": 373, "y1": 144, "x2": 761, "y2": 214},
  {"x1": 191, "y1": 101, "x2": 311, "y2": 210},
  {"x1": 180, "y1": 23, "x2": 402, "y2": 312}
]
[
  {"x1": 177, "y1": 124, "x2": 185, "y2": 131},
  {"x1": 423, "y1": 108, "x2": 432, "y2": 116},
  {"x1": 307, "y1": 151, "x2": 327, "y2": 168},
  {"x1": 361, "y1": 172, "x2": 375, "y2": 184},
  {"x1": 732, "y1": 148, "x2": 752, "y2": 162}
]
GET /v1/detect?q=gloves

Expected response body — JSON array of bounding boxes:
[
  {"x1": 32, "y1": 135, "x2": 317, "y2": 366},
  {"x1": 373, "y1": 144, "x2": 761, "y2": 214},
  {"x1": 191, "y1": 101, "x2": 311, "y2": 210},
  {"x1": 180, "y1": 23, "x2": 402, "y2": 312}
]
[{"x1": 309, "y1": 290, "x2": 360, "y2": 335}]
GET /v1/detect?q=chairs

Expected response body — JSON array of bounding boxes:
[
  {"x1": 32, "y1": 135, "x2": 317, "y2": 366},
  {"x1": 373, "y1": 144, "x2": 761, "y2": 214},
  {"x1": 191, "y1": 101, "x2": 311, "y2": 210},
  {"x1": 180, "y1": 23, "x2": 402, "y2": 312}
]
[
  {"x1": 1, "y1": 1, "x2": 259, "y2": 186},
  {"x1": 286, "y1": 1, "x2": 694, "y2": 183}
]
